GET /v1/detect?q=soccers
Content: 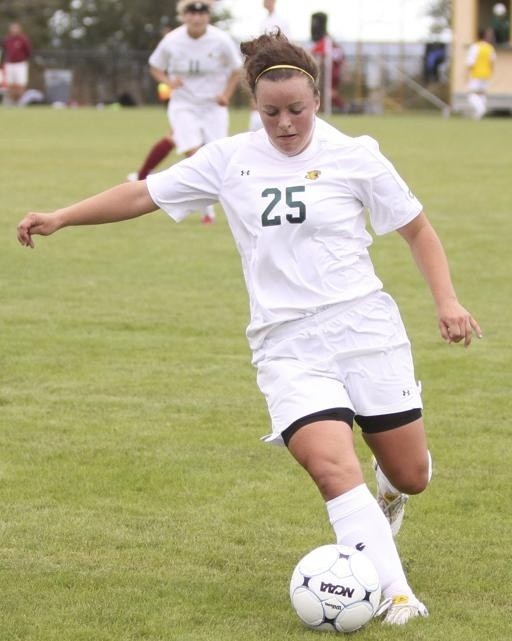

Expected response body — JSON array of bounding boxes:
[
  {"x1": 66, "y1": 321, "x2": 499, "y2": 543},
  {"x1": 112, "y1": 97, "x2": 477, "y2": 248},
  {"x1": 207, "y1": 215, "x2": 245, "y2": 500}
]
[{"x1": 289, "y1": 544, "x2": 381, "y2": 633}]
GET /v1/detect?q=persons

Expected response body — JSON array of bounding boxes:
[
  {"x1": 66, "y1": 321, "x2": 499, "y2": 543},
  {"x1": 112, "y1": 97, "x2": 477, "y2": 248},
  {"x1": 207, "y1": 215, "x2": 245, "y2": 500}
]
[
  {"x1": 15, "y1": 27, "x2": 483, "y2": 626},
  {"x1": 423, "y1": 7, "x2": 496, "y2": 120},
  {"x1": 148, "y1": 1, "x2": 242, "y2": 221},
  {"x1": 130, "y1": 133, "x2": 175, "y2": 183},
  {"x1": 314, "y1": 33, "x2": 345, "y2": 110},
  {"x1": 3, "y1": 20, "x2": 33, "y2": 105}
]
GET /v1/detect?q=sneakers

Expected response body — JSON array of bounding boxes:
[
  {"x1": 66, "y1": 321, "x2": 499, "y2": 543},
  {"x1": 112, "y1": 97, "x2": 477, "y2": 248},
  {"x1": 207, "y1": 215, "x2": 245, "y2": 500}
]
[
  {"x1": 201, "y1": 209, "x2": 215, "y2": 224},
  {"x1": 381, "y1": 595, "x2": 429, "y2": 625},
  {"x1": 372, "y1": 453, "x2": 409, "y2": 538}
]
